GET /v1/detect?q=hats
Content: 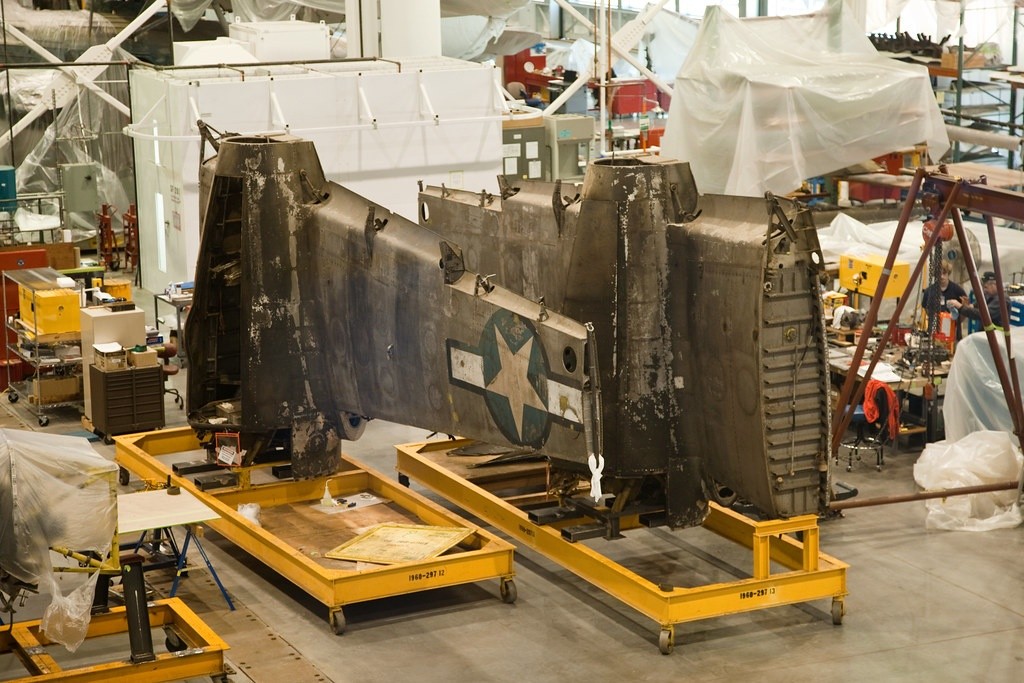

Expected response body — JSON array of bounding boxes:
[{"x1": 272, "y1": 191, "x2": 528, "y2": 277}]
[{"x1": 981, "y1": 271, "x2": 996, "y2": 282}]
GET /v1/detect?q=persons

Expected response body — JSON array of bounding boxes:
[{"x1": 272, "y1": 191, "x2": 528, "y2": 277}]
[
  {"x1": 947, "y1": 272, "x2": 1011, "y2": 330},
  {"x1": 922, "y1": 259, "x2": 969, "y2": 353}
]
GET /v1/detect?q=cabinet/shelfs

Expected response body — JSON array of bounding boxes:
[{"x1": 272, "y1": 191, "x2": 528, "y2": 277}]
[{"x1": 2, "y1": 267, "x2": 83, "y2": 426}]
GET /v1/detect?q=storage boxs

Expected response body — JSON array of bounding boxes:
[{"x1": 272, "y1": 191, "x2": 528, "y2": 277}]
[
  {"x1": 18, "y1": 284, "x2": 80, "y2": 334},
  {"x1": 840, "y1": 253, "x2": 910, "y2": 299},
  {"x1": 93, "y1": 342, "x2": 127, "y2": 371},
  {"x1": 942, "y1": 53, "x2": 985, "y2": 70},
  {"x1": 896, "y1": 423, "x2": 928, "y2": 449},
  {"x1": 33, "y1": 375, "x2": 79, "y2": 403},
  {"x1": 126, "y1": 346, "x2": 157, "y2": 368}
]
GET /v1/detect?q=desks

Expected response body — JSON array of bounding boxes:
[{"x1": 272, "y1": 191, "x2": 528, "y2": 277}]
[
  {"x1": 154, "y1": 294, "x2": 193, "y2": 368},
  {"x1": 826, "y1": 340, "x2": 952, "y2": 450},
  {"x1": 116, "y1": 487, "x2": 236, "y2": 612}
]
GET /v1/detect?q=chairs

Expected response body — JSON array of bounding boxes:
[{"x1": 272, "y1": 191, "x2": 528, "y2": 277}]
[
  {"x1": 156, "y1": 342, "x2": 183, "y2": 410},
  {"x1": 843, "y1": 381, "x2": 889, "y2": 471}
]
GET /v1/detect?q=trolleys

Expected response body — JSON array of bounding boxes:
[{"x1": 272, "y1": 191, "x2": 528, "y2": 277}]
[{"x1": 2, "y1": 266, "x2": 84, "y2": 426}]
